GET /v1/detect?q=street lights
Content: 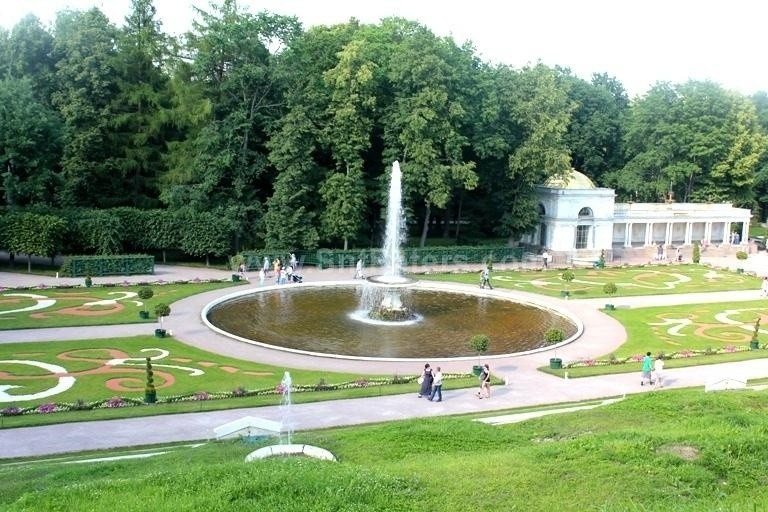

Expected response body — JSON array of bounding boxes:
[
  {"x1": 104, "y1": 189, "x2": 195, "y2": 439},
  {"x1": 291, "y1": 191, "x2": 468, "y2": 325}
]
[
  {"x1": 480, "y1": 374, "x2": 486, "y2": 380},
  {"x1": 430, "y1": 376, "x2": 433, "y2": 382}
]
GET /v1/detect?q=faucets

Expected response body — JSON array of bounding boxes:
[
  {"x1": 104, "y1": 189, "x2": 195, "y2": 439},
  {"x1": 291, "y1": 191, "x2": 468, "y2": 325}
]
[{"x1": 475, "y1": 391, "x2": 490, "y2": 399}]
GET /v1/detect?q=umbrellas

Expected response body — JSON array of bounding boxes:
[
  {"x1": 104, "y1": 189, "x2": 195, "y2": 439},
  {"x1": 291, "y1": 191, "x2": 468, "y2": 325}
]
[{"x1": 418, "y1": 394, "x2": 442, "y2": 402}]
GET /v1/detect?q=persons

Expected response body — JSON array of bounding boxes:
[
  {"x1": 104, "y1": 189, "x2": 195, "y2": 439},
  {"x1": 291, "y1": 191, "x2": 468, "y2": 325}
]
[
  {"x1": 600, "y1": 249, "x2": 605, "y2": 262},
  {"x1": 239, "y1": 261, "x2": 247, "y2": 281},
  {"x1": 479, "y1": 269, "x2": 493, "y2": 289},
  {"x1": 677, "y1": 248, "x2": 683, "y2": 261},
  {"x1": 760, "y1": 276, "x2": 768, "y2": 296},
  {"x1": 475, "y1": 364, "x2": 491, "y2": 399},
  {"x1": 418, "y1": 364, "x2": 442, "y2": 402},
  {"x1": 543, "y1": 250, "x2": 548, "y2": 268},
  {"x1": 640, "y1": 352, "x2": 664, "y2": 387},
  {"x1": 657, "y1": 246, "x2": 664, "y2": 261},
  {"x1": 353, "y1": 259, "x2": 365, "y2": 279},
  {"x1": 731, "y1": 230, "x2": 739, "y2": 244},
  {"x1": 259, "y1": 254, "x2": 296, "y2": 286}
]
